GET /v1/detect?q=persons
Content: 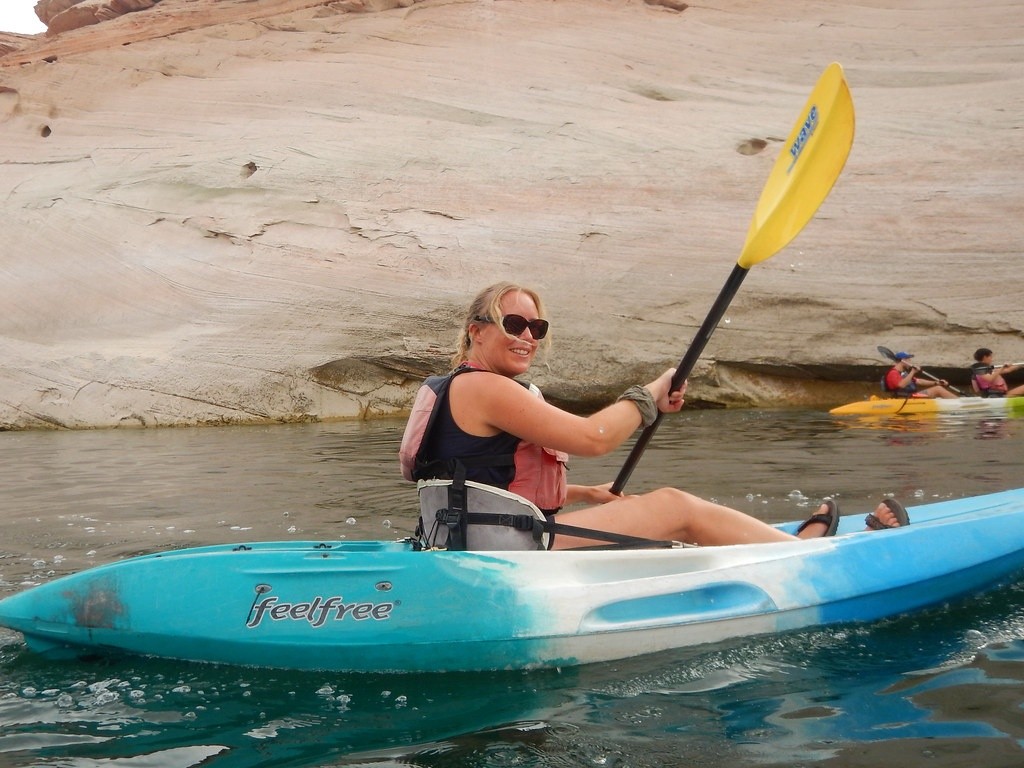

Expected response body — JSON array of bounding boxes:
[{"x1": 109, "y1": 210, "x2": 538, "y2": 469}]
[
  {"x1": 883, "y1": 352, "x2": 961, "y2": 400},
  {"x1": 968, "y1": 348, "x2": 1024, "y2": 398},
  {"x1": 397, "y1": 282, "x2": 910, "y2": 550}
]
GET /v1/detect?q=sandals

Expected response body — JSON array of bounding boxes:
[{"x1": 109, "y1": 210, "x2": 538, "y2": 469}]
[
  {"x1": 797, "y1": 499, "x2": 840, "y2": 538},
  {"x1": 864, "y1": 498, "x2": 910, "y2": 531}
]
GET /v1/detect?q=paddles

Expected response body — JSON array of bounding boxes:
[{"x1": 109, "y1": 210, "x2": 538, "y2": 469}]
[
  {"x1": 877, "y1": 345, "x2": 967, "y2": 397},
  {"x1": 608, "y1": 60, "x2": 857, "y2": 496},
  {"x1": 970, "y1": 361, "x2": 1024, "y2": 375}
]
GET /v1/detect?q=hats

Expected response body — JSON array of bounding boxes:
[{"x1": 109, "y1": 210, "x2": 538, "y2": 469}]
[{"x1": 896, "y1": 352, "x2": 914, "y2": 359}]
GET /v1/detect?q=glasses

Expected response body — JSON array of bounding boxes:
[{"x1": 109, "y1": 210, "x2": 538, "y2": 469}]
[{"x1": 475, "y1": 314, "x2": 548, "y2": 339}]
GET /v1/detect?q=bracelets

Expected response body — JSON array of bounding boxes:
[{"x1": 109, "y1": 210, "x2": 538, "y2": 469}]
[
  {"x1": 935, "y1": 381, "x2": 938, "y2": 386},
  {"x1": 615, "y1": 384, "x2": 658, "y2": 429}
]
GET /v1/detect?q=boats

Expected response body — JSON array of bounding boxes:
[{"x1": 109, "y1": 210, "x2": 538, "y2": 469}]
[
  {"x1": 829, "y1": 395, "x2": 1024, "y2": 418},
  {"x1": 0, "y1": 487, "x2": 1024, "y2": 676}
]
